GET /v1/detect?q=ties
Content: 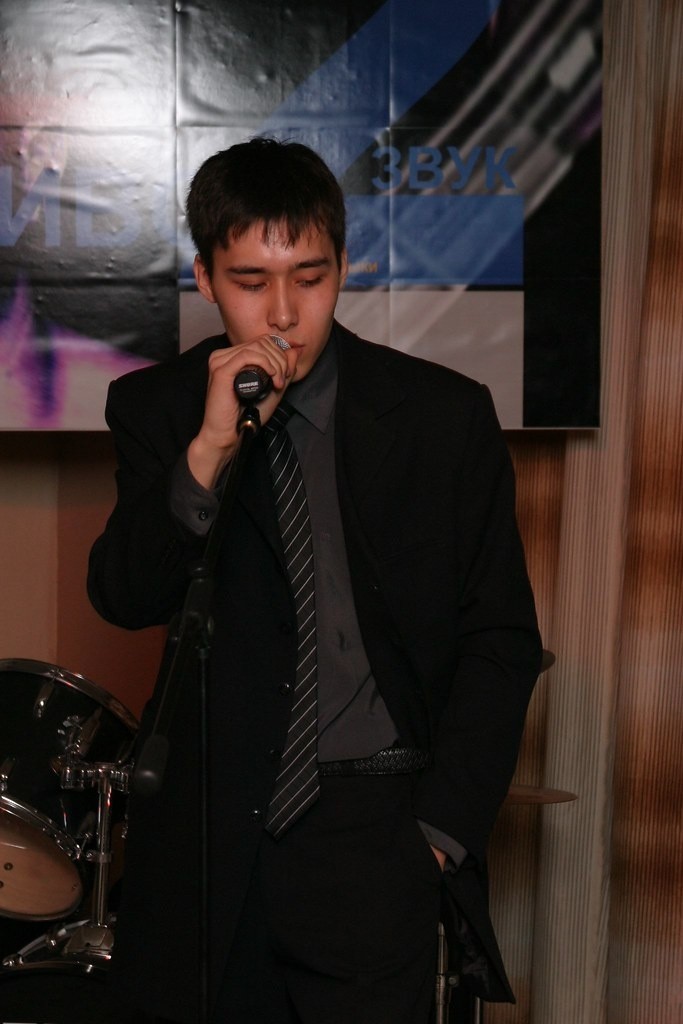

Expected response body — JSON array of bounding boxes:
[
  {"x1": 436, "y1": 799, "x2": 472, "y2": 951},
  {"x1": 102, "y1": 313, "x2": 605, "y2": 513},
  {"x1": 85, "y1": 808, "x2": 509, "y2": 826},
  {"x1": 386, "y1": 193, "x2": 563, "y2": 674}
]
[{"x1": 258, "y1": 401, "x2": 324, "y2": 852}]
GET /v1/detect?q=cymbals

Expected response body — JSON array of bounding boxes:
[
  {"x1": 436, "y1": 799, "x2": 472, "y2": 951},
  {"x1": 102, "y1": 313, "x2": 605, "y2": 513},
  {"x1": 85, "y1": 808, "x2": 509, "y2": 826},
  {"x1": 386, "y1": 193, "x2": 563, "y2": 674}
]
[{"x1": 493, "y1": 785, "x2": 581, "y2": 810}]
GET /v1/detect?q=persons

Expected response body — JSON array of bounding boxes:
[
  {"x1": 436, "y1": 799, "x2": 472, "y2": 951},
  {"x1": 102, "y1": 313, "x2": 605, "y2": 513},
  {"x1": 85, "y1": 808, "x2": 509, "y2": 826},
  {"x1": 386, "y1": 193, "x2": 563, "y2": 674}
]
[{"x1": 85, "y1": 140, "x2": 545, "y2": 1024}]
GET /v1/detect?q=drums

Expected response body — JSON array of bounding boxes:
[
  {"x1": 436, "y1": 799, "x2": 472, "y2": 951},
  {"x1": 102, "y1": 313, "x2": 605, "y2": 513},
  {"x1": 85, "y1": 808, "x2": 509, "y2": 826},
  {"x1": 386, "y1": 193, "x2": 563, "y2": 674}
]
[{"x1": 0, "y1": 658, "x2": 148, "y2": 924}]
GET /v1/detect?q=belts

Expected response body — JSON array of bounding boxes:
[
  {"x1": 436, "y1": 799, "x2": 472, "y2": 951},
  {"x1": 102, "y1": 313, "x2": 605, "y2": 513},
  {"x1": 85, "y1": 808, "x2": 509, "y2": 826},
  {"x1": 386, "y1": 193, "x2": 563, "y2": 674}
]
[{"x1": 318, "y1": 742, "x2": 433, "y2": 777}]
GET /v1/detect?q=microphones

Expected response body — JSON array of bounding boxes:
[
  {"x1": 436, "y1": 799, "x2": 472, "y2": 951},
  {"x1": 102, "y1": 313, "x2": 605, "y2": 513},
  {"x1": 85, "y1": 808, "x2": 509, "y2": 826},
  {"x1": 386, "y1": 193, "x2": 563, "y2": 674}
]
[{"x1": 234, "y1": 334, "x2": 292, "y2": 409}]
